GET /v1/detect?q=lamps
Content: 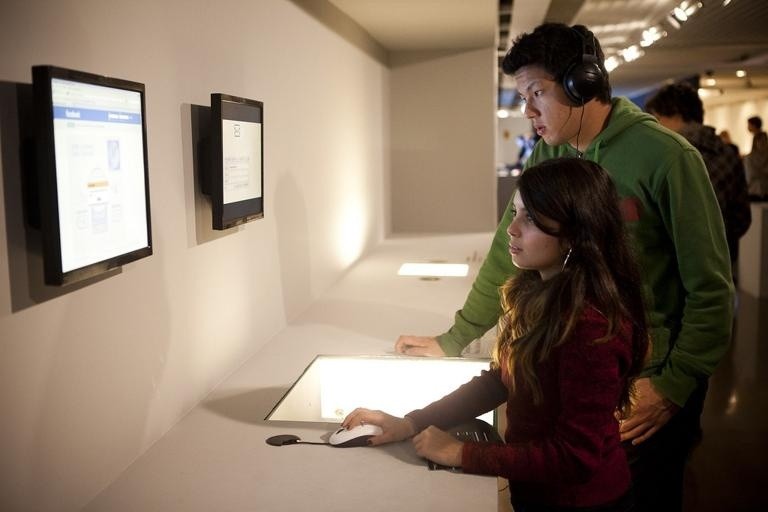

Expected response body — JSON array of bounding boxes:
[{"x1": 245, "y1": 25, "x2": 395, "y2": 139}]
[{"x1": 668, "y1": 1, "x2": 702, "y2": 29}]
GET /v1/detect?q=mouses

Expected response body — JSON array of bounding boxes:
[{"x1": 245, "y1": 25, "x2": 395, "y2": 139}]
[{"x1": 329, "y1": 423, "x2": 385, "y2": 448}]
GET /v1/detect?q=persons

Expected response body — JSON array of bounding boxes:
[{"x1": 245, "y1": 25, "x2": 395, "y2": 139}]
[
  {"x1": 717, "y1": 130, "x2": 739, "y2": 159},
  {"x1": 644, "y1": 81, "x2": 752, "y2": 419},
  {"x1": 746, "y1": 115, "x2": 767, "y2": 196},
  {"x1": 511, "y1": 125, "x2": 542, "y2": 167},
  {"x1": 341, "y1": 157, "x2": 650, "y2": 512},
  {"x1": 395, "y1": 21, "x2": 737, "y2": 512}
]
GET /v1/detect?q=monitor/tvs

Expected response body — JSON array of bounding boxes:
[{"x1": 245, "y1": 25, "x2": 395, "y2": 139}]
[
  {"x1": 211, "y1": 92, "x2": 264, "y2": 230},
  {"x1": 31, "y1": 65, "x2": 153, "y2": 286}
]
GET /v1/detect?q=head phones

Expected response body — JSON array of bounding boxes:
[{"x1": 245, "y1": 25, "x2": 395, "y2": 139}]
[{"x1": 562, "y1": 21, "x2": 605, "y2": 106}]
